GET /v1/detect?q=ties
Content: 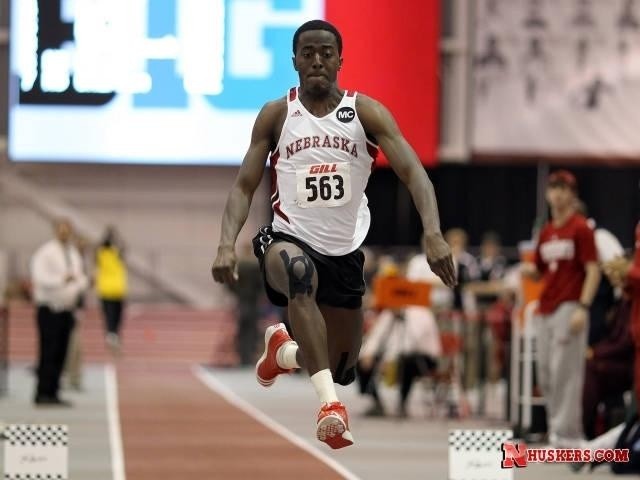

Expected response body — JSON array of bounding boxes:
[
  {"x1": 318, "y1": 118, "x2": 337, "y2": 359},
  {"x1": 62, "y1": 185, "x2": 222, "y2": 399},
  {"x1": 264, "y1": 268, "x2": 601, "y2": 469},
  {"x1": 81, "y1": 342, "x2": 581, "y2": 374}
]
[{"x1": 63, "y1": 244, "x2": 74, "y2": 275}]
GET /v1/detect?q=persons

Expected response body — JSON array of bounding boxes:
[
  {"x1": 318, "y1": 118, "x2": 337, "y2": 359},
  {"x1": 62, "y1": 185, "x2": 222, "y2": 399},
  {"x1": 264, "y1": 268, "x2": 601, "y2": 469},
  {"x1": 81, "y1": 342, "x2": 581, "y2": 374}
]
[
  {"x1": 27, "y1": 212, "x2": 134, "y2": 406},
  {"x1": 211, "y1": 19, "x2": 460, "y2": 451},
  {"x1": 527, "y1": 167, "x2": 603, "y2": 449},
  {"x1": 225, "y1": 192, "x2": 640, "y2": 452}
]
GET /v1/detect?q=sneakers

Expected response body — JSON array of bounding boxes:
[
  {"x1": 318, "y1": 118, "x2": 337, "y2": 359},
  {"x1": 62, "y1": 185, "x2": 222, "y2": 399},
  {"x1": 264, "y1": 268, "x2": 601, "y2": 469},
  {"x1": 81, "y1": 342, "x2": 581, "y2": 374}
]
[
  {"x1": 314, "y1": 401, "x2": 355, "y2": 451},
  {"x1": 546, "y1": 431, "x2": 590, "y2": 472},
  {"x1": 253, "y1": 322, "x2": 297, "y2": 390}
]
